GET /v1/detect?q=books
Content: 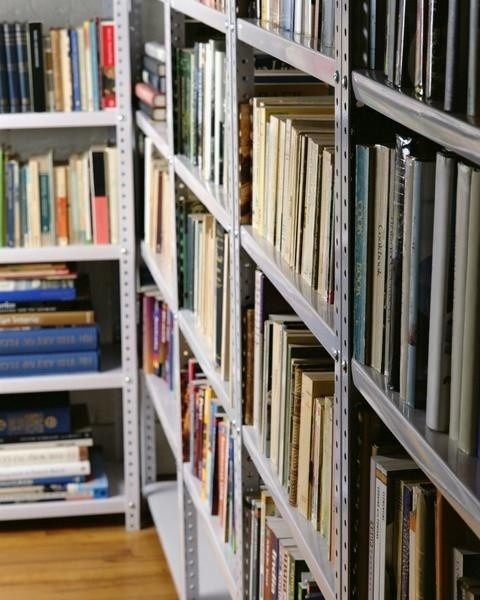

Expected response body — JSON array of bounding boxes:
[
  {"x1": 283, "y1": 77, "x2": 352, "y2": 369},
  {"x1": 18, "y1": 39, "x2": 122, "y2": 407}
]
[
  {"x1": 132, "y1": 0, "x2": 480, "y2": 600},
  {"x1": 0, "y1": 391, "x2": 108, "y2": 505},
  {"x1": 1, "y1": 264, "x2": 99, "y2": 379},
  {"x1": 0, "y1": 19, "x2": 117, "y2": 113},
  {"x1": 1, "y1": 140, "x2": 116, "y2": 248}
]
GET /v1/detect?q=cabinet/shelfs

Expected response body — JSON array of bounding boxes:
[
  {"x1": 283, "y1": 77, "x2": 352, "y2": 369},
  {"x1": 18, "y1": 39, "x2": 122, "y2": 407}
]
[
  {"x1": 1, "y1": 0, "x2": 143, "y2": 534},
  {"x1": 135, "y1": 0, "x2": 480, "y2": 599}
]
